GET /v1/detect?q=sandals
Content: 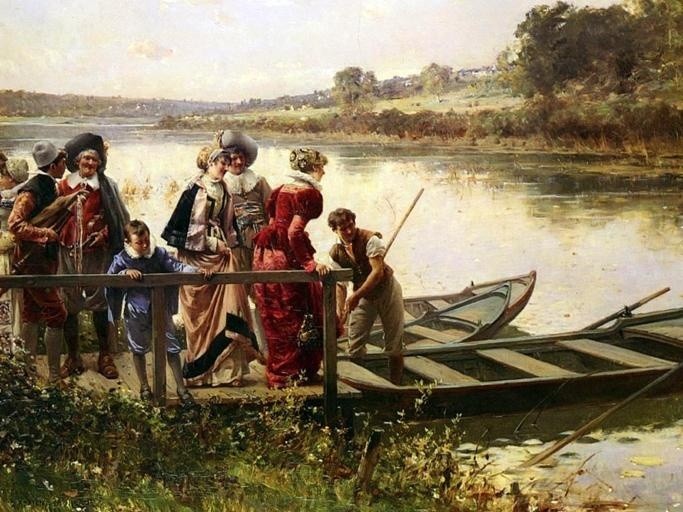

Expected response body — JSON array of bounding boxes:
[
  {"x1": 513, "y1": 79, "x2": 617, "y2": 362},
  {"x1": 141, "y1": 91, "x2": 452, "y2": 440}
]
[
  {"x1": 59, "y1": 355, "x2": 84, "y2": 379},
  {"x1": 96, "y1": 350, "x2": 119, "y2": 380}
]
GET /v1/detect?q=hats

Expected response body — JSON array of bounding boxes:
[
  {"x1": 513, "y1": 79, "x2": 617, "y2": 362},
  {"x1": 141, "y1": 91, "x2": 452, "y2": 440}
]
[
  {"x1": 64, "y1": 132, "x2": 107, "y2": 173},
  {"x1": 197, "y1": 146, "x2": 224, "y2": 170},
  {"x1": 213, "y1": 129, "x2": 259, "y2": 168},
  {"x1": 289, "y1": 149, "x2": 325, "y2": 170},
  {"x1": 4, "y1": 157, "x2": 30, "y2": 183},
  {"x1": 31, "y1": 139, "x2": 60, "y2": 169}
]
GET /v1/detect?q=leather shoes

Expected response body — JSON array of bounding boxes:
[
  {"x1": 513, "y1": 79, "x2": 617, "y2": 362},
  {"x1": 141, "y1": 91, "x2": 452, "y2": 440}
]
[
  {"x1": 177, "y1": 386, "x2": 194, "y2": 405},
  {"x1": 388, "y1": 354, "x2": 404, "y2": 386},
  {"x1": 229, "y1": 348, "x2": 324, "y2": 389},
  {"x1": 139, "y1": 386, "x2": 152, "y2": 402}
]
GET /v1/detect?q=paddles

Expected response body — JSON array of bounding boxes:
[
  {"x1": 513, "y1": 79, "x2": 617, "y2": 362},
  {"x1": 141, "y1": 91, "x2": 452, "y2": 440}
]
[{"x1": 519, "y1": 362, "x2": 682, "y2": 466}]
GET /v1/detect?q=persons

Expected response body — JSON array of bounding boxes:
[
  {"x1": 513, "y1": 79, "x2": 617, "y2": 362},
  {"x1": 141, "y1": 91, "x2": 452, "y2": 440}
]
[{"x1": 326, "y1": 209, "x2": 404, "y2": 385}]
